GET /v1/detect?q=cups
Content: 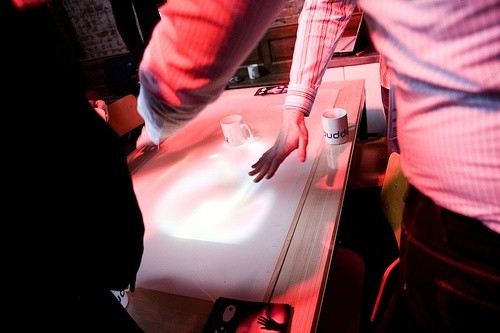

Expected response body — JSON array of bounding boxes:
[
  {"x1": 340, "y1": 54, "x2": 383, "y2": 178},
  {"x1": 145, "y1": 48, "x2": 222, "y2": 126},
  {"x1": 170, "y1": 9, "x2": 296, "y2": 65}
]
[
  {"x1": 220, "y1": 114, "x2": 253, "y2": 147},
  {"x1": 247, "y1": 64, "x2": 259, "y2": 80},
  {"x1": 321, "y1": 108, "x2": 349, "y2": 145}
]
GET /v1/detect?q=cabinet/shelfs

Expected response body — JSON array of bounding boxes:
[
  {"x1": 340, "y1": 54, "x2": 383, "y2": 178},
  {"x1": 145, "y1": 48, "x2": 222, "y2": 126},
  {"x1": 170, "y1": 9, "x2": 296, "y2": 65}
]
[{"x1": 321, "y1": 50, "x2": 387, "y2": 137}]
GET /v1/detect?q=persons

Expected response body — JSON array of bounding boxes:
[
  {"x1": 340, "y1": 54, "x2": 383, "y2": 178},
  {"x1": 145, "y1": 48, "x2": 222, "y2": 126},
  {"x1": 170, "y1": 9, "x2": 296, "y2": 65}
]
[
  {"x1": 0, "y1": 1, "x2": 145, "y2": 333},
  {"x1": 136, "y1": 0, "x2": 500, "y2": 333}
]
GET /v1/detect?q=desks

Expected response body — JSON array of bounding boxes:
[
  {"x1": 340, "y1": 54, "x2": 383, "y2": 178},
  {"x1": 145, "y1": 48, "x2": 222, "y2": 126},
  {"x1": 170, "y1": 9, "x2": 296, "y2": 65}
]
[{"x1": 111, "y1": 78, "x2": 368, "y2": 333}]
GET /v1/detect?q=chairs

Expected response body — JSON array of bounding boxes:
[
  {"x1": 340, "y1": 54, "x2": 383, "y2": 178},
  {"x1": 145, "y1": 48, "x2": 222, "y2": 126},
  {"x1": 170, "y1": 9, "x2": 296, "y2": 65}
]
[
  {"x1": 108, "y1": 94, "x2": 144, "y2": 136},
  {"x1": 370, "y1": 152, "x2": 409, "y2": 329}
]
